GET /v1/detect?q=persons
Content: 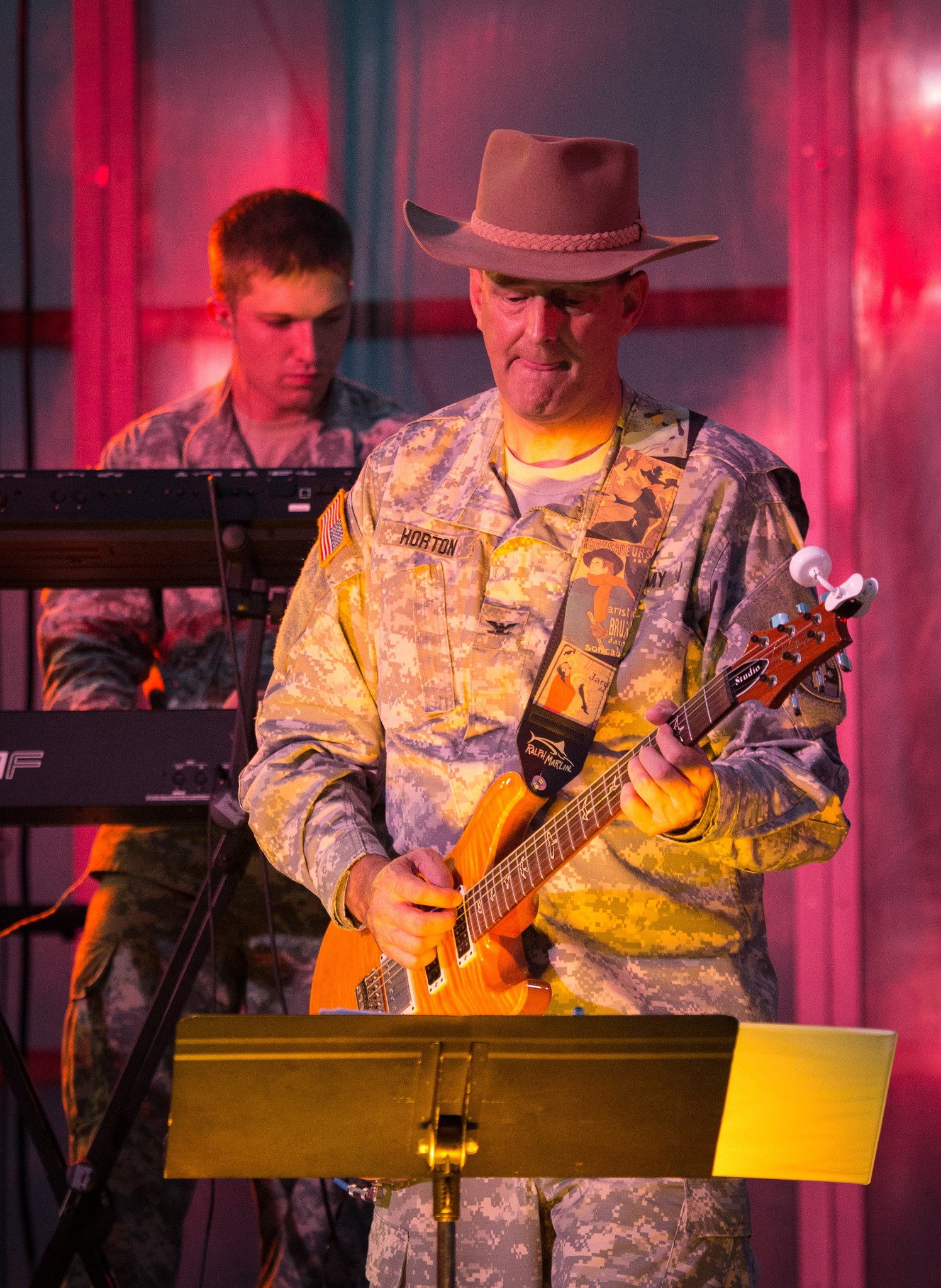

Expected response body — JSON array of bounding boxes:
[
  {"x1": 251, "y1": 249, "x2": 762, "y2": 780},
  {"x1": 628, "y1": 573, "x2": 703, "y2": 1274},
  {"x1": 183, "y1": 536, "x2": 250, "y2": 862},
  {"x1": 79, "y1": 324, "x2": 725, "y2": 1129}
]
[
  {"x1": 29, "y1": 182, "x2": 440, "y2": 1288},
  {"x1": 238, "y1": 127, "x2": 850, "y2": 1285}
]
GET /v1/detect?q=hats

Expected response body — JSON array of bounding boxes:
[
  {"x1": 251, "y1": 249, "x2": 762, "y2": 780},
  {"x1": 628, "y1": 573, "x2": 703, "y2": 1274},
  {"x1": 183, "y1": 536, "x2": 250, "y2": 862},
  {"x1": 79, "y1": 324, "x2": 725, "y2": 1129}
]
[{"x1": 402, "y1": 130, "x2": 717, "y2": 282}]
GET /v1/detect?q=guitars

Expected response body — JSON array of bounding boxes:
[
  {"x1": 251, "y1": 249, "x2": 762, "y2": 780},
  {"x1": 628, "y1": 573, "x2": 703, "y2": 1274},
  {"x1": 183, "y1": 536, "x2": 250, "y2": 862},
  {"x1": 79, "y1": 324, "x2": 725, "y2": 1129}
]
[{"x1": 303, "y1": 547, "x2": 883, "y2": 1212}]
